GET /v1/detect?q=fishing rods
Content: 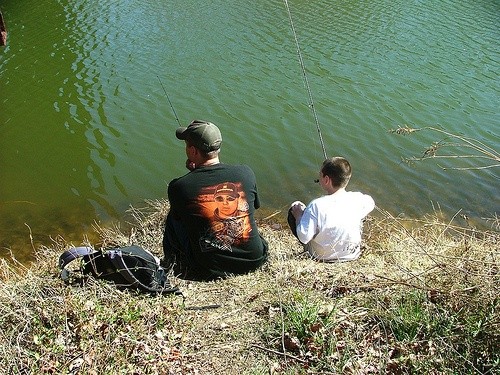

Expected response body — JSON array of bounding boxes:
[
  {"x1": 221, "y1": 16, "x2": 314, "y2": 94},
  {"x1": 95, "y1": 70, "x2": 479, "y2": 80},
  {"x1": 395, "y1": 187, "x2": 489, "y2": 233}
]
[
  {"x1": 156, "y1": 74, "x2": 181, "y2": 126},
  {"x1": 284, "y1": 0, "x2": 329, "y2": 160}
]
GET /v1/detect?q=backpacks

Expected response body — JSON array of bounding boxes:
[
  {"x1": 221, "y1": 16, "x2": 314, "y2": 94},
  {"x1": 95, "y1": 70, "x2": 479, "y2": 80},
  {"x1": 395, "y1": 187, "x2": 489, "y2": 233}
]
[{"x1": 58, "y1": 245, "x2": 181, "y2": 295}]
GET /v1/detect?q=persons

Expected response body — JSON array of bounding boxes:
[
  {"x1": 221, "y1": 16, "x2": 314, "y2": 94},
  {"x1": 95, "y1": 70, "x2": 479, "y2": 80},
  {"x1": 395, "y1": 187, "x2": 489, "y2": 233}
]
[
  {"x1": 287, "y1": 157, "x2": 375, "y2": 264},
  {"x1": 163, "y1": 119, "x2": 269, "y2": 280},
  {"x1": 209, "y1": 182, "x2": 251, "y2": 239}
]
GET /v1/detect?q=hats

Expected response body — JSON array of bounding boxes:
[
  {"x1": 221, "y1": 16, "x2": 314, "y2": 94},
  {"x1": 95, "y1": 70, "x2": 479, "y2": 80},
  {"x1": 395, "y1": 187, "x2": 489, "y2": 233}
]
[{"x1": 176, "y1": 120, "x2": 223, "y2": 155}]
